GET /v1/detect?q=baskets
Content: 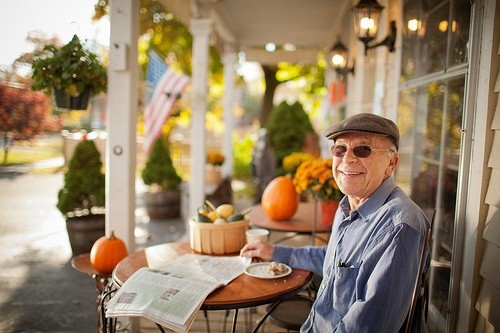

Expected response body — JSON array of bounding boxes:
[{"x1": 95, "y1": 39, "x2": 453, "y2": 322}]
[{"x1": 187, "y1": 216, "x2": 250, "y2": 256}]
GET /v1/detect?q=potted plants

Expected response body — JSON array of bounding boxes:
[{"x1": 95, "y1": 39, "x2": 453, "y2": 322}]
[
  {"x1": 56, "y1": 137, "x2": 105, "y2": 256},
  {"x1": 142, "y1": 138, "x2": 183, "y2": 220},
  {"x1": 30, "y1": 33, "x2": 107, "y2": 110}
]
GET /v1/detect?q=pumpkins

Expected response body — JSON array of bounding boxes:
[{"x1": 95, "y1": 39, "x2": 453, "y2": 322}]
[
  {"x1": 261, "y1": 172, "x2": 298, "y2": 220},
  {"x1": 199, "y1": 200, "x2": 252, "y2": 224},
  {"x1": 90, "y1": 230, "x2": 128, "y2": 274}
]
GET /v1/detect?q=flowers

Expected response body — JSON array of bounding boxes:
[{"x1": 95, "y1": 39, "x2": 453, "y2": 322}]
[{"x1": 290, "y1": 159, "x2": 343, "y2": 197}]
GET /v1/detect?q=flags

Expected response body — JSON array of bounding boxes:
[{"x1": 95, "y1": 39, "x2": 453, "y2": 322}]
[{"x1": 142, "y1": 50, "x2": 193, "y2": 157}]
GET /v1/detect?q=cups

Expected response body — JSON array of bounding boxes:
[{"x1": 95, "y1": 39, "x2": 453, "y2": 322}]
[{"x1": 245, "y1": 228, "x2": 270, "y2": 244}]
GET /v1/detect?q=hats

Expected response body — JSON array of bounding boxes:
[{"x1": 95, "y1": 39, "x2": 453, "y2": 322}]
[{"x1": 324, "y1": 112, "x2": 400, "y2": 152}]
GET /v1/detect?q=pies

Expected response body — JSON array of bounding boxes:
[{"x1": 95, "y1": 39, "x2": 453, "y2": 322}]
[{"x1": 267, "y1": 261, "x2": 289, "y2": 276}]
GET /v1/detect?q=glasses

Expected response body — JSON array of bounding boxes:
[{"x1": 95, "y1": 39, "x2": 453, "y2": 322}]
[{"x1": 328, "y1": 144, "x2": 390, "y2": 158}]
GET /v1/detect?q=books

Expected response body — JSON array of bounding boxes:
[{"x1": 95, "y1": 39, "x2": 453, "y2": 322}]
[{"x1": 104, "y1": 254, "x2": 253, "y2": 333}]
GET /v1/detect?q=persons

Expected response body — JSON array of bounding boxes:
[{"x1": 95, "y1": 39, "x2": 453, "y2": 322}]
[{"x1": 240, "y1": 113, "x2": 431, "y2": 333}]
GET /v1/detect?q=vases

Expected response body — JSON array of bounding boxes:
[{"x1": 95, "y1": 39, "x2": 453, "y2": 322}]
[{"x1": 323, "y1": 197, "x2": 339, "y2": 228}]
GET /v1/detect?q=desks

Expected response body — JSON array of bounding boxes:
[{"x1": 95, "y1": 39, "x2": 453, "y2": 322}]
[
  {"x1": 246, "y1": 200, "x2": 330, "y2": 245},
  {"x1": 112, "y1": 242, "x2": 310, "y2": 333}
]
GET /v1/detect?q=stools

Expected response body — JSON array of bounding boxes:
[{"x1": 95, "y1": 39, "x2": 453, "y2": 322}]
[
  {"x1": 264, "y1": 296, "x2": 317, "y2": 333},
  {"x1": 71, "y1": 251, "x2": 112, "y2": 333}
]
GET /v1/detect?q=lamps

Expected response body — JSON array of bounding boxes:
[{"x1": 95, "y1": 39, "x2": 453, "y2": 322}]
[
  {"x1": 330, "y1": 36, "x2": 354, "y2": 79},
  {"x1": 350, "y1": 0, "x2": 397, "y2": 56}
]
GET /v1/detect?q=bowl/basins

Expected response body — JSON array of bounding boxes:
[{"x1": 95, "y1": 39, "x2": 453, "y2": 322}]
[{"x1": 189, "y1": 214, "x2": 250, "y2": 255}]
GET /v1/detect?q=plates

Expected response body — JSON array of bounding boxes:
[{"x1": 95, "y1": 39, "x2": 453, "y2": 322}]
[{"x1": 242, "y1": 261, "x2": 292, "y2": 279}]
